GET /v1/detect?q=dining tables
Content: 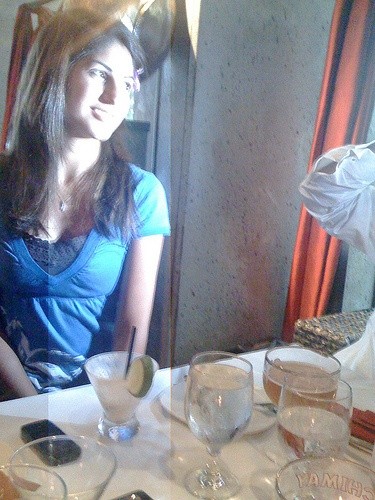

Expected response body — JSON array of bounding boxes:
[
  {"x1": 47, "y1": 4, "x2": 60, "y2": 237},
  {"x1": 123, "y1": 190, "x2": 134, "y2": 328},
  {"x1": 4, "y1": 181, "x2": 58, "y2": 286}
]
[{"x1": 0, "y1": 346, "x2": 375, "y2": 500}]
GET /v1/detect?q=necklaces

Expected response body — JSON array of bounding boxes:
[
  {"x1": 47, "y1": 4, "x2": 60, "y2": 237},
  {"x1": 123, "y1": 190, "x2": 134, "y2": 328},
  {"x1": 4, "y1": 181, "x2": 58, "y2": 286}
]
[{"x1": 50, "y1": 182, "x2": 82, "y2": 212}]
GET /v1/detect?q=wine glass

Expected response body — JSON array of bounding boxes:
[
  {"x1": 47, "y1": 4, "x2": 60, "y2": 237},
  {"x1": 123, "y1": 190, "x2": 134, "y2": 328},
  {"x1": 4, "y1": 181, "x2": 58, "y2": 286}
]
[
  {"x1": 262, "y1": 346, "x2": 343, "y2": 466},
  {"x1": 183, "y1": 350, "x2": 254, "y2": 500}
]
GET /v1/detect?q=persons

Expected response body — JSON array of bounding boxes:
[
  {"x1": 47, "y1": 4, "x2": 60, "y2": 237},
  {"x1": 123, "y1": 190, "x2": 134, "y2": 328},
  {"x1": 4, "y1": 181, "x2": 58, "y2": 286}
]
[
  {"x1": 299, "y1": 140, "x2": 375, "y2": 378},
  {"x1": 0, "y1": 9, "x2": 171, "y2": 401}
]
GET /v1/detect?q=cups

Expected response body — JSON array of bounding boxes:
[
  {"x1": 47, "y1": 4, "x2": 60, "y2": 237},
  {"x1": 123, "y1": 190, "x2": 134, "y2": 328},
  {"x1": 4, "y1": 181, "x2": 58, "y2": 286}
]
[
  {"x1": 0, "y1": 462, "x2": 67, "y2": 500},
  {"x1": 83, "y1": 350, "x2": 160, "y2": 442},
  {"x1": 275, "y1": 455, "x2": 375, "y2": 500},
  {"x1": 7, "y1": 434, "x2": 118, "y2": 500},
  {"x1": 275, "y1": 373, "x2": 353, "y2": 460}
]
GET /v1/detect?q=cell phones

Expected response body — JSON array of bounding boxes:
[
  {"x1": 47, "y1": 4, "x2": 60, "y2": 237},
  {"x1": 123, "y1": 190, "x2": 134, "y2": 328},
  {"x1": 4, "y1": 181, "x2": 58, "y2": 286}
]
[{"x1": 21, "y1": 420, "x2": 81, "y2": 468}]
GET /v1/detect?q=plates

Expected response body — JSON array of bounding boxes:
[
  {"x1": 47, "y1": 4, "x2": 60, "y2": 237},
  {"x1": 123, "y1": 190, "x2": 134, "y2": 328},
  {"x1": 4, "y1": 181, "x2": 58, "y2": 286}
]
[{"x1": 155, "y1": 374, "x2": 278, "y2": 435}]
